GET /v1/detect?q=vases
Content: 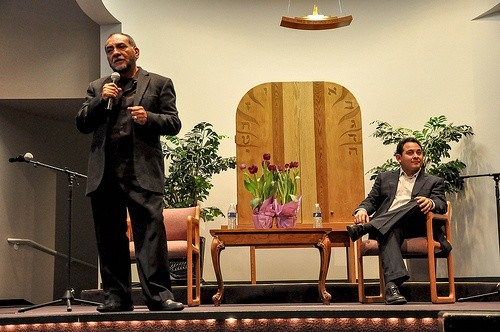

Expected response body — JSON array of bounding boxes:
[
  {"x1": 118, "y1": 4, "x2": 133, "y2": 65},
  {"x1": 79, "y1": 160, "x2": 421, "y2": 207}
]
[
  {"x1": 252, "y1": 215, "x2": 273, "y2": 229},
  {"x1": 277, "y1": 216, "x2": 297, "y2": 229}
]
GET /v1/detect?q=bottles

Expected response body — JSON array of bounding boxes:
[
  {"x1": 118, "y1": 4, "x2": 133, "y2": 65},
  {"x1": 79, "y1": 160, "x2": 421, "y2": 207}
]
[
  {"x1": 312, "y1": 203, "x2": 322, "y2": 228},
  {"x1": 227, "y1": 204, "x2": 236, "y2": 230}
]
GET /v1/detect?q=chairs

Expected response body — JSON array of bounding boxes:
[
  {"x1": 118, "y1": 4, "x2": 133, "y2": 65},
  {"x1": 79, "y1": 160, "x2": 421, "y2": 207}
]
[
  {"x1": 127, "y1": 205, "x2": 201, "y2": 306},
  {"x1": 357, "y1": 201, "x2": 455, "y2": 304}
]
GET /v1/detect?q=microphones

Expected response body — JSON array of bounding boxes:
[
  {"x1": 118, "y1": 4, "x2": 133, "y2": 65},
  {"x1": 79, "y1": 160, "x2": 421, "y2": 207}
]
[
  {"x1": 8, "y1": 152, "x2": 34, "y2": 163},
  {"x1": 105, "y1": 72, "x2": 121, "y2": 111}
]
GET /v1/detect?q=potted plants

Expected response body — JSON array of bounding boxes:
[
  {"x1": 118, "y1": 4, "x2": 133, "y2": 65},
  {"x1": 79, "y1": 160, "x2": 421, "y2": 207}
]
[{"x1": 364, "y1": 115, "x2": 475, "y2": 282}]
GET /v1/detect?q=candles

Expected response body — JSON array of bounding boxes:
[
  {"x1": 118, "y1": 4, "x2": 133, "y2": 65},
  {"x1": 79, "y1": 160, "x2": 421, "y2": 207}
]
[{"x1": 161, "y1": 122, "x2": 237, "y2": 286}]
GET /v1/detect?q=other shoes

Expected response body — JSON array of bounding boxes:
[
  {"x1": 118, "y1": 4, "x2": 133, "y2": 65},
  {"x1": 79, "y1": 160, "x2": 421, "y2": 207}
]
[
  {"x1": 149, "y1": 299, "x2": 184, "y2": 311},
  {"x1": 97, "y1": 299, "x2": 135, "y2": 312},
  {"x1": 345, "y1": 224, "x2": 366, "y2": 243},
  {"x1": 384, "y1": 286, "x2": 408, "y2": 304}
]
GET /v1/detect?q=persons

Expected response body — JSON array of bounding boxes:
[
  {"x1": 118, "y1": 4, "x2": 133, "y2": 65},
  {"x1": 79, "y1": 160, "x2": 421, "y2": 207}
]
[
  {"x1": 347, "y1": 137, "x2": 448, "y2": 305},
  {"x1": 76, "y1": 33, "x2": 185, "y2": 312}
]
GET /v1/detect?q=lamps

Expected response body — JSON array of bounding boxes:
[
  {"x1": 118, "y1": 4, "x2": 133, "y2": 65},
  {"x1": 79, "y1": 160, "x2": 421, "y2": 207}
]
[{"x1": 280, "y1": 0, "x2": 353, "y2": 30}]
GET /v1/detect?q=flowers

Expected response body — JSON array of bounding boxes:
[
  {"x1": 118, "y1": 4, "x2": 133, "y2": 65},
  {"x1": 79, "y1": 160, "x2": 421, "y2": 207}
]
[{"x1": 240, "y1": 153, "x2": 302, "y2": 218}]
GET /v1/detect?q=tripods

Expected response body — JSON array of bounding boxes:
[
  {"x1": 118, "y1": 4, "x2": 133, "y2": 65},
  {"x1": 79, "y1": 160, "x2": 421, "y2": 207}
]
[{"x1": 18, "y1": 160, "x2": 103, "y2": 312}]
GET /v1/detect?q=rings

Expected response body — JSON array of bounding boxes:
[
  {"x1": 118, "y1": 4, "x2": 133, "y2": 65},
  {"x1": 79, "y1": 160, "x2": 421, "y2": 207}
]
[{"x1": 135, "y1": 116, "x2": 137, "y2": 119}]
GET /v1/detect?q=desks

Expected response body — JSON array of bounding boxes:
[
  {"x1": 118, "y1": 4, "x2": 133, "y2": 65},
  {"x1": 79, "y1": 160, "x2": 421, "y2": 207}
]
[
  {"x1": 210, "y1": 228, "x2": 332, "y2": 307},
  {"x1": 221, "y1": 222, "x2": 355, "y2": 285}
]
